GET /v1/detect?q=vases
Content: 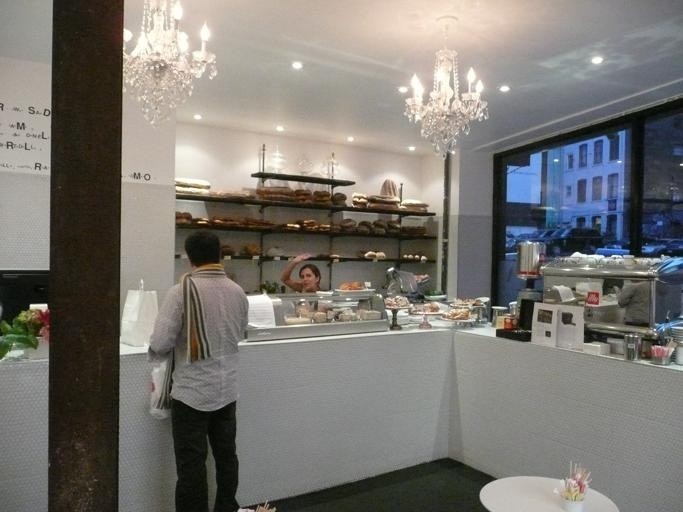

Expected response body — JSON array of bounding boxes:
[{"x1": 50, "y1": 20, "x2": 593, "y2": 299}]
[{"x1": 23, "y1": 336, "x2": 49, "y2": 360}]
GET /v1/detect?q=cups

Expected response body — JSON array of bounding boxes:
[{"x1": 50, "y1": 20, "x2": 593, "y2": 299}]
[{"x1": 623, "y1": 334, "x2": 643, "y2": 361}]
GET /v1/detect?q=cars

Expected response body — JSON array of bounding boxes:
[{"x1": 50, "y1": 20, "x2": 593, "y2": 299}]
[
  {"x1": 642, "y1": 238, "x2": 683, "y2": 258},
  {"x1": 603, "y1": 241, "x2": 630, "y2": 250}
]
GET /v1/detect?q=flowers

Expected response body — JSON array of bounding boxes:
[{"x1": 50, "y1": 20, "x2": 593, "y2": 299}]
[{"x1": 0, "y1": 307, "x2": 50, "y2": 360}]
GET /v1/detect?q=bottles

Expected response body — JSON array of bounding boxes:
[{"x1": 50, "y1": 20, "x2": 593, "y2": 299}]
[{"x1": 491, "y1": 301, "x2": 518, "y2": 330}]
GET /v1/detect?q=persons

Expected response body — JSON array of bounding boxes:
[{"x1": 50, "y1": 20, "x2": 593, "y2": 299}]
[
  {"x1": 145, "y1": 230, "x2": 250, "y2": 512},
  {"x1": 279, "y1": 252, "x2": 330, "y2": 313}
]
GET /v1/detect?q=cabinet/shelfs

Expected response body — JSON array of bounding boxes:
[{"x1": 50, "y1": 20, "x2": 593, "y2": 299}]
[
  {"x1": 245, "y1": 288, "x2": 390, "y2": 342},
  {"x1": 175, "y1": 144, "x2": 437, "y2": 295}
]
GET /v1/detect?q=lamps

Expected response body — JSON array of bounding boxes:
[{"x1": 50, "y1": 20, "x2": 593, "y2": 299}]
[
  {"x1": 515, "y1": 240, "x2": 546, "y2": 331},
  {"x1": 404, "y1": 16, "x2": 488, "y2": 163},
  {"x1": 122, "y1": 1, "x2": 219, "y2": 127}
]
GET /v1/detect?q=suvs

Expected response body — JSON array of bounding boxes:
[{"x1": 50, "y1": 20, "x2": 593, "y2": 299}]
[{"x1": 506, "y1": 228, "x2": 602, "y2": 258}]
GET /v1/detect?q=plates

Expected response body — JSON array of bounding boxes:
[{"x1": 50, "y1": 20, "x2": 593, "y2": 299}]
[{"x1": 425, "y1": 294, "x2": 445, "y2": 302}]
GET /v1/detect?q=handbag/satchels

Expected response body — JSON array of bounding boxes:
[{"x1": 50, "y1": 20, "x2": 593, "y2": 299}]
[{"x1": 150, "y1": 364, "x2": 170, "y2": 420}]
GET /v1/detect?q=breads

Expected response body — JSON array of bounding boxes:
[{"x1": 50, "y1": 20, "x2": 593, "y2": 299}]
[{"x1": 175, "y1": 176, "x2": 428, "y2": 260}]
